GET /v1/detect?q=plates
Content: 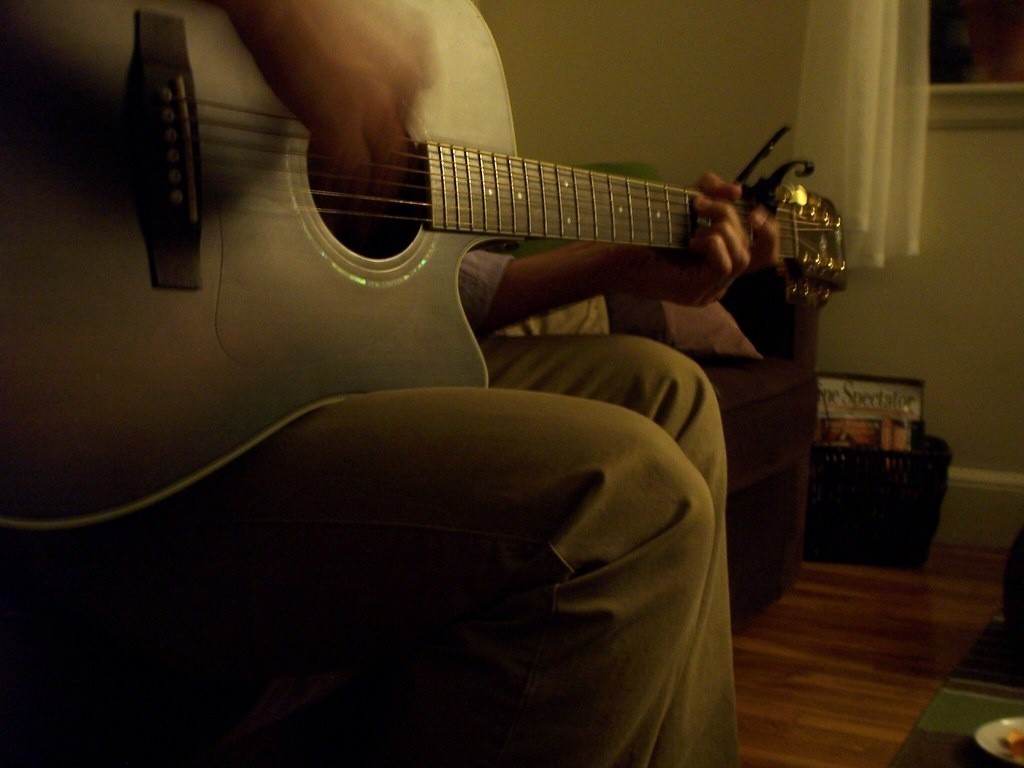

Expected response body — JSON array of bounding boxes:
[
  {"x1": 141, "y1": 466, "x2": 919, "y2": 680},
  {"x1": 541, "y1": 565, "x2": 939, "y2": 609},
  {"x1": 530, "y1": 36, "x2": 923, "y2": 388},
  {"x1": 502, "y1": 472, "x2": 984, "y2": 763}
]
[{"x1": 974, "y1": 715, "x2": 1024, "y2": 766}]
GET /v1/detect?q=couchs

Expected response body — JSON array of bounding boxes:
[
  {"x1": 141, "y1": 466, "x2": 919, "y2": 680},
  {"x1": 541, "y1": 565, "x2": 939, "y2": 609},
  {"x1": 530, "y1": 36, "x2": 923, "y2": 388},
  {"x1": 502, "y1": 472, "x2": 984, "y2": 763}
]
[{"x1": 482, "y1": 163, "x2": 839, "y2": 596}]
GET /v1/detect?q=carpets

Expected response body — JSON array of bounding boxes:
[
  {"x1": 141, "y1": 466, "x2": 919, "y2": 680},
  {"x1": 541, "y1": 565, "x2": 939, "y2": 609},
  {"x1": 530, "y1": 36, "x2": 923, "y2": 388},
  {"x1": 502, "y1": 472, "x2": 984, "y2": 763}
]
[{"x1": 889, "y1": 622, "x2": 1024, "y2": 768}]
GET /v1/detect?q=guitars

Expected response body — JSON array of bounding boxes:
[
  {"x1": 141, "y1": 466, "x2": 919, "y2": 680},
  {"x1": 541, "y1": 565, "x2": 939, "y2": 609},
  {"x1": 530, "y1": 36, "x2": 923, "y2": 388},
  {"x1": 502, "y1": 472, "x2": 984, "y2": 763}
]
[{"x1": 0, "y1": 0, "x2": 849, "y2": 531}]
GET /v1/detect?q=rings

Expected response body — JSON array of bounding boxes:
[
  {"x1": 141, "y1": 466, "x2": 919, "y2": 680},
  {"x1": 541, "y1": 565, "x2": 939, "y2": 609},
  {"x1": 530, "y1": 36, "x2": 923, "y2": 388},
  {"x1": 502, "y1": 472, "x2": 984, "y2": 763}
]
[{"x1": 718, "y1": 280, "x2": 732, "y2": 287}]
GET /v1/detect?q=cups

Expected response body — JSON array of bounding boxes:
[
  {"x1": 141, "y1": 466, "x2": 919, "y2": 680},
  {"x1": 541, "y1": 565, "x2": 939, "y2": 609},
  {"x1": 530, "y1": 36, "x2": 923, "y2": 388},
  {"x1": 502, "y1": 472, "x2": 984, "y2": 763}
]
[{"x1": 882, "y1": 413, "x2": 911, "y2": 451}]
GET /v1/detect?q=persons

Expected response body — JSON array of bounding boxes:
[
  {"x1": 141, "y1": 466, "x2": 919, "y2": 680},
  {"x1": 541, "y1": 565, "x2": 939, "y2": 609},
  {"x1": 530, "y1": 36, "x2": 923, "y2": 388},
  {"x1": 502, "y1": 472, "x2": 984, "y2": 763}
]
[{"x1": 92, "y1": 0, "x2": 780, "y2": 768}]
